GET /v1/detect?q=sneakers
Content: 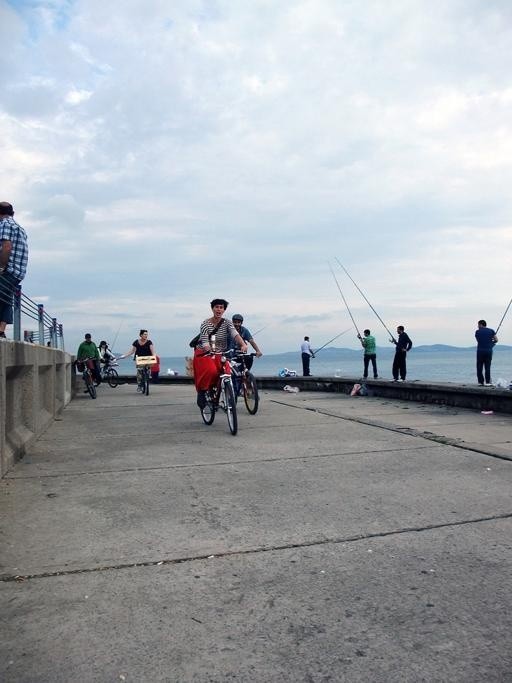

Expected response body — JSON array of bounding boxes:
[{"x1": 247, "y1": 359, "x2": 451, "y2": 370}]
[
  {"x1": 485, "y1": 383, "x2": 495, "y2": 387},
  {"x1": 138, "y1": 385, "x2": 142, "y2": 392},
  {"x1": 397, "y1": 378, "x2": 407, "y2": 382},
  {"x1": 196, "y1": 393, "x2": 206, "y2": 409},
  {"x1": 389, "y1": 379, "x2": 397, "y2": 381},
  {"x1": 477, "y1": 382, "x2": 484, "y2": 386},
  {"x1": 221, "y1": 406, "x2": 232, "y2": 414},
  {"x1": 149, "y1": 375, "x2": 153, "y2": 379}
]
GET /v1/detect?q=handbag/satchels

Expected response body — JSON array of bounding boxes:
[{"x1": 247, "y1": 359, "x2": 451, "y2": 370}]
[{"x1": 190, "y1": 318, "x2": 226, "y2": 348}]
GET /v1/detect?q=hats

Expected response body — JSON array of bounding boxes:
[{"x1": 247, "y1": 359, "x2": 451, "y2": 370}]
[
  {"x1": 233, "y1": 313, "x2": 243, "y2": 322},
  {"x1": 101, "y1": 341, "x2": 106, "y2": 345},
  {"x1": 85, "y1": 334, "x2": 91, "y2": 339}
]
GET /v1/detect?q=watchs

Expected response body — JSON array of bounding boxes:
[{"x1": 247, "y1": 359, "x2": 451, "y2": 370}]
[{"x1": 0, "y1": 268, "x2": 4, "y2": 272}]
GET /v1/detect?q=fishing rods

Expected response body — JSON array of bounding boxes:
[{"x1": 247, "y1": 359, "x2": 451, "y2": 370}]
[
  {"x1": 335, "y1": 257, "x2": 398, "y2": 344},
  {"x1": 328, "y1": 262, "x2": 363, "y2": 345},
  {"x1": 495, "y1": 300, "x2": 512, "y2": 334},
  {"x1": 311, "y1": 329, "x2": 352, "y2": 356}
]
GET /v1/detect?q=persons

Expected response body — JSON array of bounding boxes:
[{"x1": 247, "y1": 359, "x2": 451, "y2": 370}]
[
  {"x1": 97, "y1": 341, "x2": 116, "y2": 374},
  {"x1": 301, "y1": 336, "x2": 315, "y2": 376},
  {"x1": 150, "y1": 355, "x2": 160, "y2": 384},
  {"x1": 47, "y1": 341, "x2": 51, "y2": 347},
  {"x1": 356, "y1": 329, "x2": 378, "y2": 379},
  {"x1": 121, "y1": 329, "x2": 156, "y2": 392},
  {"x1": 388, "y1": 325, "x2": 412, "y2": 383},
  {"x1": 76, "y1": 333, "x2": 103, "y2": 384},
  {"x1": 474, "y1": 319, "x2": 498, "y2": 387},
  {"x1": 192, "y1": 298, "x2": 248, "y2": 414},
  {"x1": 225, "y1": 314, "x2": 263, "y2": 411},
  {"x1": 0, "y1": 202, "x2": 28, "y2": 339}
]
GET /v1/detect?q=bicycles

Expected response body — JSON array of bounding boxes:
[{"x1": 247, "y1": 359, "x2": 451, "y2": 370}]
[
  {"x1": 200, "y1": 347, "x2": 247, "y2": 440},
  {"x1": 91, "y1": 357, "x2": 121, "y2": 389},
  {"x1": 135, "y1": 362, "x2": 162, "y2": 396},
  {"x1": 74, "y1": 356, "x2": 100, "y2": 400},
  {"x1": 220, "y1": 351, "x2": 259, "y2": 416}
]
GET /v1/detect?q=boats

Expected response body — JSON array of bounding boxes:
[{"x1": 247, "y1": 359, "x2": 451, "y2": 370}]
[{"x1": 161, "y1": 367, "x2": 182, "y2": 376}]
